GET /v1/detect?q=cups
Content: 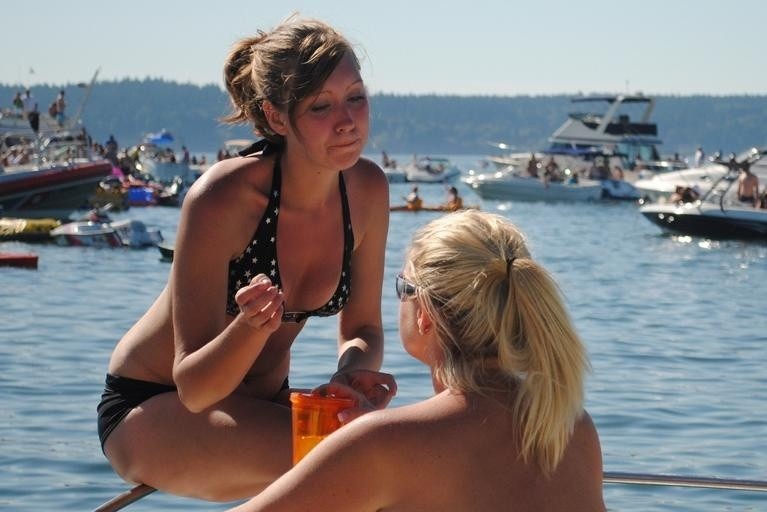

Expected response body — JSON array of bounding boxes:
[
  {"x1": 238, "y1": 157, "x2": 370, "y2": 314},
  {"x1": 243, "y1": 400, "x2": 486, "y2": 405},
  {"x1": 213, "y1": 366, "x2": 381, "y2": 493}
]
[{"x1": 289, "y1": 392, "x2": 359, "y2": 466}]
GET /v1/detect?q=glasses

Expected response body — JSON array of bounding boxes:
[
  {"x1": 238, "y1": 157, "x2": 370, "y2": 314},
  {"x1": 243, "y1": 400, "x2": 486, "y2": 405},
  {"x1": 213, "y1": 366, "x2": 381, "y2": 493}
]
[{"x1": 393, "y1": 270, "x2": 421, "y2": 302}]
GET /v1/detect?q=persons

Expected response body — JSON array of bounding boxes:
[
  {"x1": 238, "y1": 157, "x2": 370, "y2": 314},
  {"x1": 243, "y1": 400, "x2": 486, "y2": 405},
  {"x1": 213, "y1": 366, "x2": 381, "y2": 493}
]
[
  {"x1": 223, "y1": 209, "x2": 610, "y2": 512},
  {"x1": 97, "y1": 8, "x2": 397, "y2": 503}
]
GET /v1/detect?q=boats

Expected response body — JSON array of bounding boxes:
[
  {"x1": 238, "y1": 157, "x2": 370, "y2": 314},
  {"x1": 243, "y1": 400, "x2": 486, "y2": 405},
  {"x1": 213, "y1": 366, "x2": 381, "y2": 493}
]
[
  {"x1": 383, "y1": 155, "x2": 459, "y2": 183},
  {"x1": 388, "y1": 202, "x2": 478, "y2": 215}
]
